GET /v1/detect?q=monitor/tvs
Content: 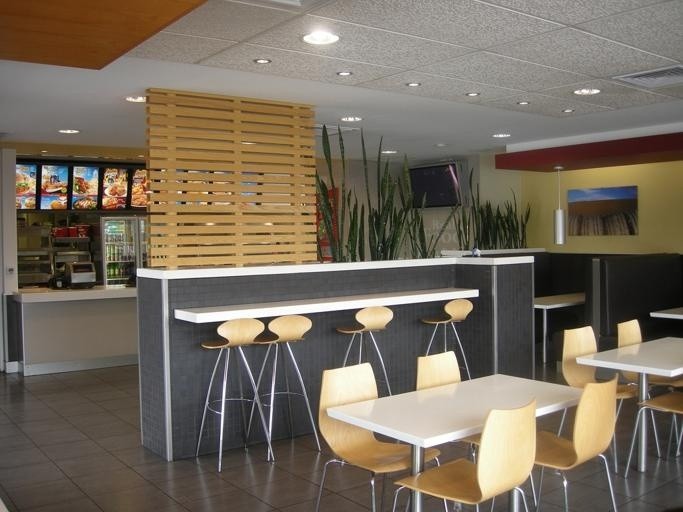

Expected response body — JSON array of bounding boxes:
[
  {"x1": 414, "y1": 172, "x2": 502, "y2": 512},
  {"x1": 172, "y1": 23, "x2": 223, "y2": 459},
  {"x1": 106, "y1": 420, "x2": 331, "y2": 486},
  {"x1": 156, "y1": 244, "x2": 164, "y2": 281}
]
[{"x1": 408, "y1": 162, "x2": 462, "y2": 208}]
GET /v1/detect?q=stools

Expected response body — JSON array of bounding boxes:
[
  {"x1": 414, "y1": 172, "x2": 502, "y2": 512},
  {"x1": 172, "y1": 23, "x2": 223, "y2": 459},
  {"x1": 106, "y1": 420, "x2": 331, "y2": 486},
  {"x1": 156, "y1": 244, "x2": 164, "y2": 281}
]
[
  {"x1": 196, "y1": 318, "x2": 275, "y2": 472},
  {"x1": 337, "y1": 306, "x2": 394, "y2": 399},
  {"x1": 247, "y1": 316, "x2": 321, "y2": 461},
  {"x1": 422, "y1": 299, "x2": 474, "y2": 379}
]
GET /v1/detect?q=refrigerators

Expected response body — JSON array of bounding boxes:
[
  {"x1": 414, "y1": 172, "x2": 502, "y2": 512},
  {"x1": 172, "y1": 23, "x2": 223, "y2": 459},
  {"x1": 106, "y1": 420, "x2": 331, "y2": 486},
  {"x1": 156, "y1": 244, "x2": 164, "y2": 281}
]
[
  {"x1": 89, "y1": 215, "x2": 139, "y2": 290},
  {"x1": 140, "y1": 216, "x2": 146, "y2": 266}
]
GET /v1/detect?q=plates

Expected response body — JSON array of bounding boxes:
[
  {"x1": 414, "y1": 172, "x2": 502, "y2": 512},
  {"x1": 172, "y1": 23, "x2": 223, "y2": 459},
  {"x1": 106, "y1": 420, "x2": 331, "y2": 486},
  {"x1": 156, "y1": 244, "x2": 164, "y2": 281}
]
[
  {"x1": 104, "y1": 185, "x2": 127, "y2": 197},
  {"x1": 130, "y1": 195, "x2": 146, "y2": 208}
]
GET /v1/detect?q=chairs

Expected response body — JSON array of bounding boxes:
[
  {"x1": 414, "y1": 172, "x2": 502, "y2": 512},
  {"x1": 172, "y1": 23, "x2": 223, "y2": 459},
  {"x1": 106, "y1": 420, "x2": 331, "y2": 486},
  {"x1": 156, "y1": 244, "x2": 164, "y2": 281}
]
[
  {"x1": 626, "y1": 393, "x2": 682, "y2": 480},
  {"x1": 314, "y1": 363, "x2": 448, "y2": 512},
  {"x1": 417, "y1": 352, "x2": 481, "y2": 460},
  {"x1": 535, "y1": 372, "x2": 620, "y2": 509},
  {"x1": 395, "y1": 398, "x2": 537, "y2": 512},
  {"x1": 556, "y1": 326, "x2": 661, "y2": 474},
  {"x1": 613, "y1": 320, "x2": 682, "y2": 448}
]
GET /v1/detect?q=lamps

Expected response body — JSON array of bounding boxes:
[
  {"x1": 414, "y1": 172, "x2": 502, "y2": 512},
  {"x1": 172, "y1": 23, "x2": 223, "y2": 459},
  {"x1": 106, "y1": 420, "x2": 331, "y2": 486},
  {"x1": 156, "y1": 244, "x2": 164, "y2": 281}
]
[{"x1": 552, "y1": 166, "x2": 566, "y2": 245}]
